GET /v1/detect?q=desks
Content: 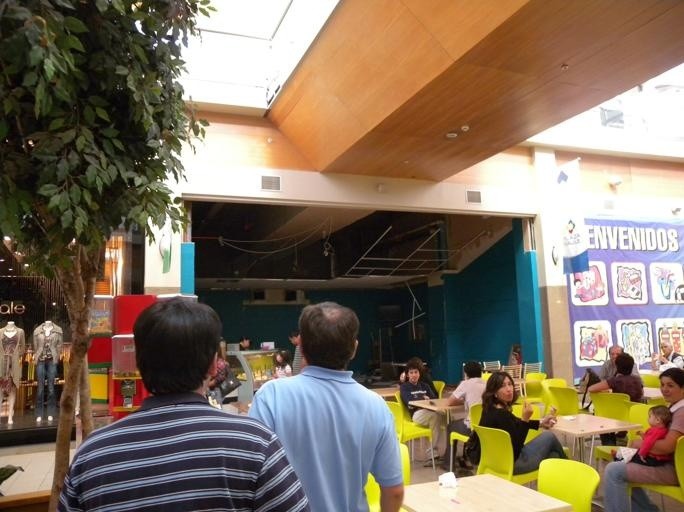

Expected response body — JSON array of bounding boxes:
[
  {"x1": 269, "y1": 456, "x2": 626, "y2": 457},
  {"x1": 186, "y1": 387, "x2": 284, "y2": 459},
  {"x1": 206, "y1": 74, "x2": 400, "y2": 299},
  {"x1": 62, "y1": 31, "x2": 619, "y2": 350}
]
[
  {"x1": 369, "y1": 387, "x2": 399, "y2": 399},
  {"x1": 566, "y1": 384, "x2": 588, "y2": 393},
  {"x1": 401, "y1": 474, "x2": 573, "y2": 512},
  {"x1": 540, "y1": 414, "x2": 642, "y2": 463},
  {"x1": 408, "y1": 398, "x2": 466, "y2": 470},
  {"x1": 640, "y1": 387, "x2": 664, "y2": 403}
]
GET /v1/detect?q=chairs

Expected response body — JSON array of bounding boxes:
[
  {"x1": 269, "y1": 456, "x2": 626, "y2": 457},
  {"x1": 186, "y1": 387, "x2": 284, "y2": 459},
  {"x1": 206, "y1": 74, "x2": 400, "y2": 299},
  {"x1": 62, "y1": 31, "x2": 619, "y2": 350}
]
[
  {"x1": 402, "y1": 419, "x2": 438, "y2": 472},
  {"x1": 385, "y1": 402, "x2": 403, "y2": 443},
  {"x1": 513, "y1": 396, "x2": 543, "y2": 405},
  {"x1": 472, "y1": 424, "x2": 539, "y2": 484},
  {"x1": 550, "y1": 387, "x2": 593, "y2": 416},
  {"x1": 538, "y1": 458, "x2": 600, "y2": 512},
  {"x1": 484, "y1": 361, "x2": 500, "y2": 374},
  {"x1": 481, "y1": 373, "x2": 493, "y2": 380},
  {"x1": 648, "y1": 397, "x2": 669, "y2": 407},
  {"x1": 624, "y1": 399, "x2": 645, "y2": 408},
  {"x1": 594, "y1": 405, "x2": 657, "y2": 496},
  {"x1": 523, "y1": 362, "x2": 542, "y2": 395},
  {"x1": 525, "y1": 372, "x2": 547, "y2": 381},
  {"x1": 364, "y1": 444, "x2": 410, "y2": 511},
  {"x1": 589, "y1": 392, "x2": 630, "y2": 423},
  {"x1": 450, "y1": 403, "x2": 483, "y2": 471},
  {"x1": 462, "y1": 362, "x2": 481, "y2": 381},
  {"x1": 396, "y1": 391, "x2": 446, "y2": 448},
  {"x1": 433, "y1": 381, "x2": 445, "y2": 400},
  {"x1": 509, "y1": 405, "x2": 543, "y2": 444},
  {"x1": 501, "y1": 365, "x2": 522, "y2": 395},
  {"x1": 639, "y1": 374, "x2": 662, "y2": 387},
  {"x1": 542, "y1": 378, "x2": 567, "y2": 391},
  {"x1": 524, "y1": 382, "x2": 552, "y2": 415},
  {"x1": 626, "y1": 437, "x2": 684, "y2": 512}
]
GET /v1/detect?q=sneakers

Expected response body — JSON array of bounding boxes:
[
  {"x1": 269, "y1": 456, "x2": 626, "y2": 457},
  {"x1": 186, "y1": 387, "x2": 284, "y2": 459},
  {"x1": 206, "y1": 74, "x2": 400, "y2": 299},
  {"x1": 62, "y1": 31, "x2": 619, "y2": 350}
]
[
  {"x1": 461, "y1": 459, "x2": 472, "y2": 469},
  {"x1": 440, "y1": 463, "x2": 455, "y2": 472},
  {"x1": 423, "y1": 460, "x2": 433, "y2": 466},
  {"x1": 426, "y1": 448, "x2": 439, "y2": 459}
]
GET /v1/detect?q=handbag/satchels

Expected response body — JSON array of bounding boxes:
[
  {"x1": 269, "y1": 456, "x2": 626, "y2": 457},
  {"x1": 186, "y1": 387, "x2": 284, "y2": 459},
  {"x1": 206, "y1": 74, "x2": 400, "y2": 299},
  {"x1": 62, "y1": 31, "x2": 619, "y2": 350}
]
[
  {"x1": 464, "y1": 431, "x2": 481, "y2": 466},
  {"x1": 579, "y1": 366, "x2": 601, "y2": 393},
  {"x1": 219, "y1": 370, "x2": 243, "y2": 398}
]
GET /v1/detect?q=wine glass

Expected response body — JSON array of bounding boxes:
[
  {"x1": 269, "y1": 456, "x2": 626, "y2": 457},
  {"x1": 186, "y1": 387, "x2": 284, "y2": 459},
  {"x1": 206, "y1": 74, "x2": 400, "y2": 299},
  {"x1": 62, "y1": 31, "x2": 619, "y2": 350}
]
[{"x1": 433, "y1": 380, "x2": 445, "y2": 404}]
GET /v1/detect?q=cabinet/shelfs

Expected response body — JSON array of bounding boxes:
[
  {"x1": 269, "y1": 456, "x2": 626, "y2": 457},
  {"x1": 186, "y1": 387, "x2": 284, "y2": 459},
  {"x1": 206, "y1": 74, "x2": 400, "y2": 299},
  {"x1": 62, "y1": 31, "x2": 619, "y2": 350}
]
[
  {"x1": 226, "y1": 348, "x2": 279, "y2": 402},
  {"x1": 108, "y1": 294, "x2": 200, "y2": 422}
]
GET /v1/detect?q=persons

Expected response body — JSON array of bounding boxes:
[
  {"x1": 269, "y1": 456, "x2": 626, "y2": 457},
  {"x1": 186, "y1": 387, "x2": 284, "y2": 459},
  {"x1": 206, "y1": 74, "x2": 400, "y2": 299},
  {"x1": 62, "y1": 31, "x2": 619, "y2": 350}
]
[
  {"x1": 612, "y1": 405, "x2": 672, "y2": 467},
  {"x1": 602, "y1": 367, "x2": 684, "y2": 512},
  {"x1": 588, "y1": 353, "x2": 644, "y2": 462},
  {"x1": 270, "y1": 349, "x2": 292, "y2": 380},
  {"x1": 398, "y1": 344, "x2": 569, "y2": 475},
  {"x1": 652, "y1": 340, "x2": 684, "y2": 372},
  {"x1": 288, "y1": 328, "x2": 308, "y2": 376},
  {"x1": 247, "y1": 301, "x2": 404, "y2": 512},
  {"x1": 32, "y1": 320, "x2": 64, "y2": 422},
  {"x1": 0, "y1": 321, "x2": 26, "y2": 425},
  {"x1": 237, "y1": 334, "x2": 250, "y2": 352},
  {"x1": 203, "y1": 356, "x2": 229, "y2": 409},
  {"x1": 600, "y1": 345, "x2": 640, "y2": 385},
  {"x1": 58, "y1": 297, "x2": 313, "y2": 512}
]
